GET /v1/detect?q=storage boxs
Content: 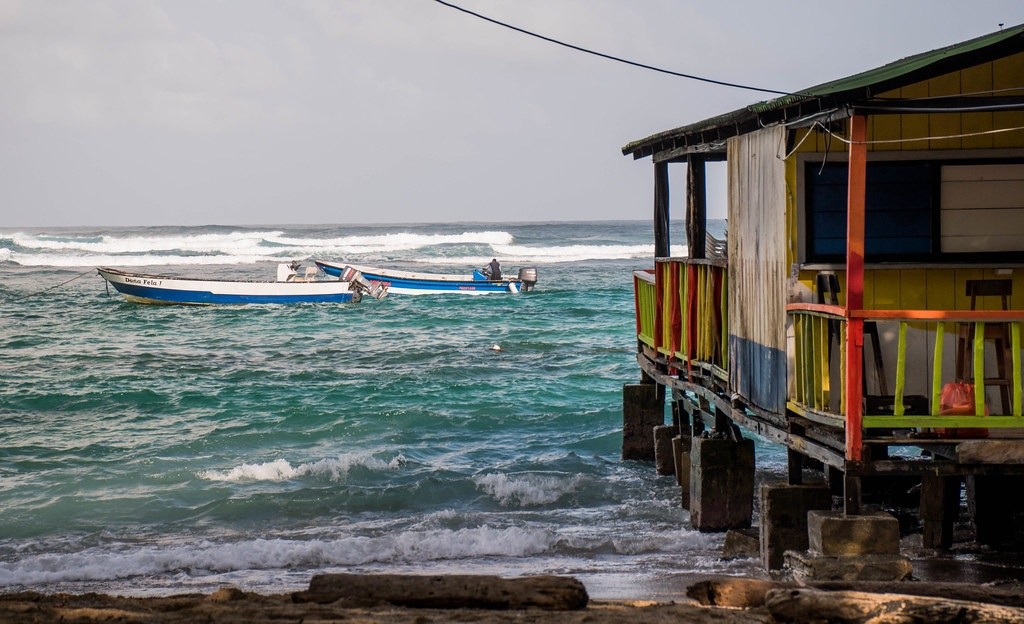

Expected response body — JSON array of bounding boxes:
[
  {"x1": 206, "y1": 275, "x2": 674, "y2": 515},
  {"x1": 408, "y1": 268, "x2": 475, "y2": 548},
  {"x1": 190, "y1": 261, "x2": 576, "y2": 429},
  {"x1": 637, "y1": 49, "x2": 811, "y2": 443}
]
[{"x1": 861, "y1": 395, "x2": 929, "y2": 436}]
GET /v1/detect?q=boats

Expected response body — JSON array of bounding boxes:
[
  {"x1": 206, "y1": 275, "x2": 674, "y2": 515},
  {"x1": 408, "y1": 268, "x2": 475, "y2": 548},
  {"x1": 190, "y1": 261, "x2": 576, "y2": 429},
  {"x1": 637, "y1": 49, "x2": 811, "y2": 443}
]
[
  {"x1": 315, "y1": 259, "x2": 538, "y2": 293},
  {"x1": 96, "y1": 265, "x2": 389, "y2": 306}
]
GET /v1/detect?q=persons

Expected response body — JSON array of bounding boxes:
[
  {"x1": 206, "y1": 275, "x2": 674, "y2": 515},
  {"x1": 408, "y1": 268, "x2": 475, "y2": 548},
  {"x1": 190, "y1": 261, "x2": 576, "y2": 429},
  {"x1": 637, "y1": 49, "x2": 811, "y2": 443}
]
[{"x1": 485, "y1": 258, "x2": 501, "y2": 280}]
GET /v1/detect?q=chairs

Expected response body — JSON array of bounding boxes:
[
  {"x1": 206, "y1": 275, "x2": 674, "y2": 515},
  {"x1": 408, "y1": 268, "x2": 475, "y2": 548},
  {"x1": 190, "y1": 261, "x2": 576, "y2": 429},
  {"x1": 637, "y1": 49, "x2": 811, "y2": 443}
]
[
  {"x1": 956, "y1": 279, "x2": 1014, "y2": 416},
  {"x1": 816, "y1": 270, "x2": 890, "y2": 413},
  {"x1": 705, "y1": 218, "x2": 728, "y2": 260},
  {"x1": 304, "y1": 267, "x2": 317, "y2": 280}
]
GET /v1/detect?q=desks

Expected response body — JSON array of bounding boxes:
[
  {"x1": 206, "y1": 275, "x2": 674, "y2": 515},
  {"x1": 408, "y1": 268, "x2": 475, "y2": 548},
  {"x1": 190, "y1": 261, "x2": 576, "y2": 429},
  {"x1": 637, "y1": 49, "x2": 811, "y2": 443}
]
[{"x1": 894, "y1": 318, "x2": 1023, "y2": 416}]
